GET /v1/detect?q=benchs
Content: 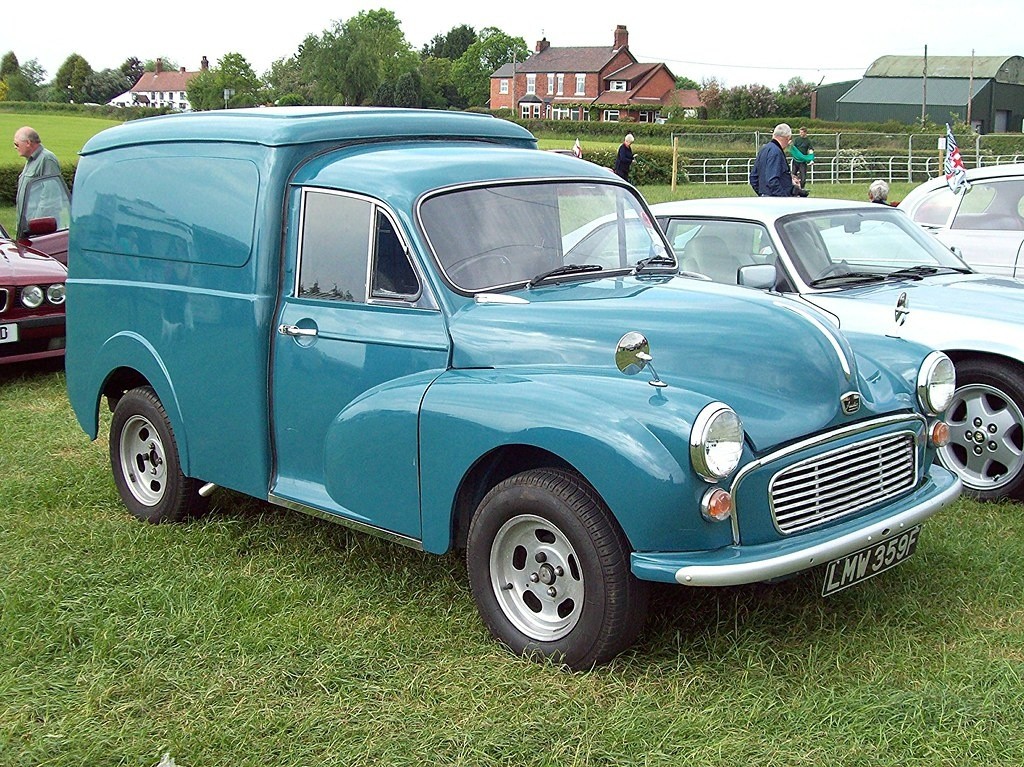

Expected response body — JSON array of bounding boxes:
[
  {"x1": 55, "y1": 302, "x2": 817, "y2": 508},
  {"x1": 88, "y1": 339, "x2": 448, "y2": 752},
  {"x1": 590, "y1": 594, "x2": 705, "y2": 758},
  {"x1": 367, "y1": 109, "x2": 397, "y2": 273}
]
[{"x1": 924, "y1": 212, "x2": 1021, "y2": 230}]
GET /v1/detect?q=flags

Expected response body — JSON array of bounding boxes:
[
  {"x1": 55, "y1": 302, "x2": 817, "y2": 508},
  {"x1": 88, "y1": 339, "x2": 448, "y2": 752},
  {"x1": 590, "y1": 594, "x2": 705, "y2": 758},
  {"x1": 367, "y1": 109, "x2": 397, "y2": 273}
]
[
  {"x1": 945, "y1": 123, "x2": 972, "y2": 194},
  {"x1": 573, "y1": 138, "x2": 584, "y2": 158}
]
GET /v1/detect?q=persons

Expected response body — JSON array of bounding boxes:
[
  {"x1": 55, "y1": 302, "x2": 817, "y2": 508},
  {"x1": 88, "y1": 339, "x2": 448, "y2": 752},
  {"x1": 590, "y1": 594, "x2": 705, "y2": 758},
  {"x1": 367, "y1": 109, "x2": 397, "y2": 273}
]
[
  {"x1": 788, "y1": 126, "x2": 814, "y2": 191},
  {"x1": 868, "y1": 179, "x2": 890, "y2": 207},
  {"x1": 12, "y1": 125, "x2": 72, "y2": 242},
  {"x1": 615, "y1": 133, "x2": 640, "y2": 183},
  {"x1": 749, "y1": 123, "x2": 810, "y2": 198}
]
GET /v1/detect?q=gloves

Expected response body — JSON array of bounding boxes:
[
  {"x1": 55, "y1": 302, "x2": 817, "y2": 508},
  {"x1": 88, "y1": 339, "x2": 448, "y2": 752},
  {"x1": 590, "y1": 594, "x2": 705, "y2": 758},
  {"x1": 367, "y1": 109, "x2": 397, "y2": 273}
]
[{"x1": 792, "y1": 185, "x2": 811, "y2": 197}]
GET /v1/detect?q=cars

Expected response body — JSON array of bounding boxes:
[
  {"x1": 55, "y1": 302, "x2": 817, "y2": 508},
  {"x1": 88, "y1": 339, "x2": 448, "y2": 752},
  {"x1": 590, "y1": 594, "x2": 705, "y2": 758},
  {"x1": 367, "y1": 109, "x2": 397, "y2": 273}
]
[
  {"x1": 0, "y1": 217, "x2": 69, "y2": 365},
  {"x1": 893, "y1": 163, "x2": 1024, "y2": 276},
  {"x1": 558, "y1": 196, "x2": 1024, "y2": 499}
]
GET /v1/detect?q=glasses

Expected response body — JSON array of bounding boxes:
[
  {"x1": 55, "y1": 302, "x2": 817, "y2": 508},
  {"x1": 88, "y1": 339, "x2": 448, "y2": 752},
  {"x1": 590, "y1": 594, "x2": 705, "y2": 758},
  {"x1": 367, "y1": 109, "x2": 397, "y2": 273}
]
[{"x1": 14, "y1": 143, "x2": 20, "y2": 147}]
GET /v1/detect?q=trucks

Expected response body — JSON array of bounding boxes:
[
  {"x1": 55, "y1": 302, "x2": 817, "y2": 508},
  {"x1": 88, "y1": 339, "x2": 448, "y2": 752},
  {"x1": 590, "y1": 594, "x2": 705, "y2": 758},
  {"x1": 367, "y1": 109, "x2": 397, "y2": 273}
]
[{"x1": 62, "y1": 104, "x2": 962, "y2": 673}]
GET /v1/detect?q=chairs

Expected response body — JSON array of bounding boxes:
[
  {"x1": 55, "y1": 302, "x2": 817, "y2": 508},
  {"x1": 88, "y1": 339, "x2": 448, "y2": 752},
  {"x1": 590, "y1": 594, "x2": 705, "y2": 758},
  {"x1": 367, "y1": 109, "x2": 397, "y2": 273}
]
[
  {"x1": 677, "y1": 235, "x2": 736, "y2": 283},
  {"x1": 774, "y1": 228, "x2": 834, "y2": 290}
]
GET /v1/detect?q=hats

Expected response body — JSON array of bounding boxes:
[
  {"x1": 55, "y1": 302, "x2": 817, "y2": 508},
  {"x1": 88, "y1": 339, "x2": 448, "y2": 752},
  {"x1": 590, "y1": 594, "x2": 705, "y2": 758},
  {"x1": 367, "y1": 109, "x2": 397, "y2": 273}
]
[{"x1": 870, "y1": 180, "x2": 889, "y2": 201}]
